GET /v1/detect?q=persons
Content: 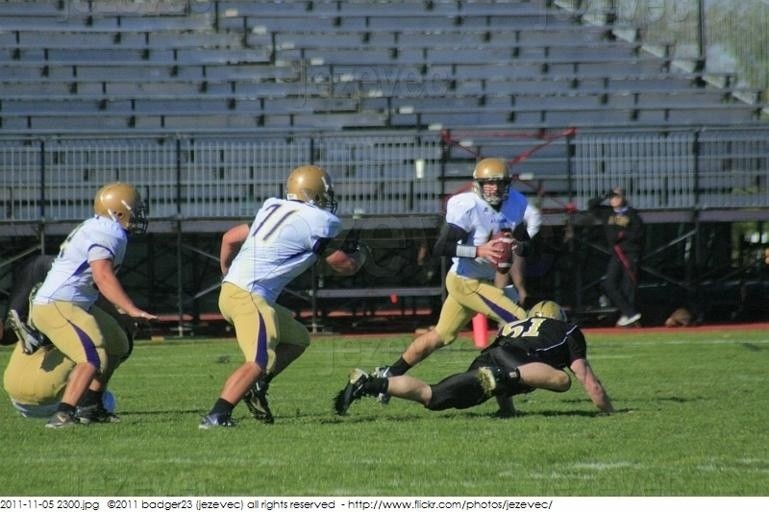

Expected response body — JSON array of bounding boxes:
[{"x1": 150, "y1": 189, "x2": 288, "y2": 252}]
[
  {"x1": 199, "y1": 165, "x2": 373, "y2": 428},
  {"x1": 4, "y1": 181, "x2": 159, "y2": 430},
  {"x1": 589, "y1": 188, "x2": 647, "y2": 329},
  {"x1": 335, "y1": 299, "x2": 616, "y2": 416},
  {"x1": 494, "y1": 239, "x2": 528, "y2": 305},
  {"x1": 374, "y1": 156, "x2": 542, "y2": 402}
]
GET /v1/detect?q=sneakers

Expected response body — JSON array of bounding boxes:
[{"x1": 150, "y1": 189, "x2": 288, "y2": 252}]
[
  {"x1": 74, "y1": 402, "x2": 123, "y2": 425},
  {"x1": 371, "y1": 363, "x2": 396, "y2": 406},
  {"x1": 7, "y1": 310, "x2": 44, "y2": 354},
  {"x1": 243, "y1": 381, "x2": 274, "y2": 426},
  {"x1": 477, "y1": 365, "x2": 504, "y2": 395},
  {"x1": 199, "y1": 414, "x2": 239, "y2": 430},
  {"x1": 25, "y1": 282, "x2": 46, "y2": 334},
  {"x1": 333, "y1": 366, "x2": 373, "y2": 416},
  {"x1": 44, "y1": 410, "x2": 87, "y2": 429},
  {"x1": 617, "y1": 313, "x2": 642, "y2": 327},
  {"x1": 495, "y1": 391, "x2": 518, "y2": 413}
]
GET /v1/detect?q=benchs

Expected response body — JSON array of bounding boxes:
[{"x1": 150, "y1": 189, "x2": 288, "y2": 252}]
[{"x1": 308, "y1": 283, "x2": 443, "y2": 329}]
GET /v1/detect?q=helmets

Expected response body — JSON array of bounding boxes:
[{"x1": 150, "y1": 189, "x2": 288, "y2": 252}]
[
  {"x1": 94, "y1": 182, "x2": 148, "y2": 241},
  {"x1": 472, "y1": 158, "x2": 512, "y2": 205},
  {"x1": 285, "y1": 165, "x2": 338, "y2": 217},
  {"x1": 527, "y1": 300, "x2": 566, "y2": 323}
]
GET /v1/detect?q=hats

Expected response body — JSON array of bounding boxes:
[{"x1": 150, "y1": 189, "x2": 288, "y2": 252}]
[{"x1": 609, "y1": 188, "x2": 627, "y2": 200}]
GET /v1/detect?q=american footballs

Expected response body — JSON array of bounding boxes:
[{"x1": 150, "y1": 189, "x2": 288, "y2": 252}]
[{"x1": 492, "y1": 231, "x2": 513, "y2": 274}]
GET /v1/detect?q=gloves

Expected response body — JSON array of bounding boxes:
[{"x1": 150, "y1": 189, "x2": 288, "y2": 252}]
[
  {"x1": 606, "y1": 230, "x2": 619, "y2": 247},
  {"x1": 509, "y1": 239, "x2": 525, "y2": 258},
  {"x1": 601, "y1": 189, "x2": 615, "y2": 200}
]
